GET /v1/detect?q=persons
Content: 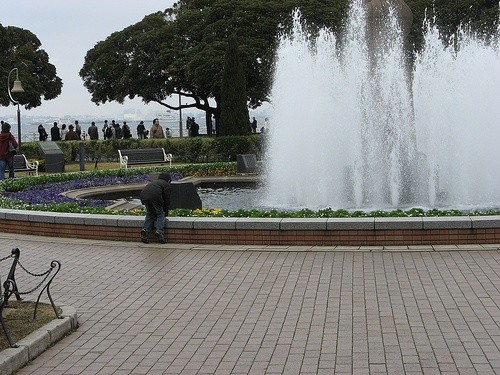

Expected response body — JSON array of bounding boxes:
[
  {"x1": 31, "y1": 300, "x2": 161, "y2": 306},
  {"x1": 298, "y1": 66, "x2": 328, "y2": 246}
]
[
  {"x1": 140, "y1": 172, "x2": 172, "y2": 244},
  {"x1": 50, "y1": 120, "x2": 82, "y2": 142},
  {"x1": 136, "y1": 119, "x2": 171, "y2": 139},
  {"x1": 186, "y1": 117, "x2": 200, "y2": 136},
  {"x1": 0, "y1": 122, "x2": 18, "y2": 180},
  {"x1": 88, "y1": 122, "x2": 98, "y2": 140},
  {"x1": 38, "y1": 124, "x2": 47, "y2": 141},
  {"x1": 102, "y1": 120, "x2": 131, "y2": 141},
  {"x1": 251, "y1": 117, "x2": 256, "y2": 132}
]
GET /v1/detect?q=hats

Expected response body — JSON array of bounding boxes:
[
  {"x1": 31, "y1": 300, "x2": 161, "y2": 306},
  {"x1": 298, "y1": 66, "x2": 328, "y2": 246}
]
[{"x1": 1, "y1": 121, "x2": 11, "y2": 131}]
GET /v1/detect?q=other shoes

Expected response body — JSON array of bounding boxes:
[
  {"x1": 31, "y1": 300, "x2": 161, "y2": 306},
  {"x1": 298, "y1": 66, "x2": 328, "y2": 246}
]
[
  {"x1": 155, "y1": 231, "x2": 166, "y2": 243},
  {"x1": 141, "y1": 229, "x2": 148, "y2": 244}
]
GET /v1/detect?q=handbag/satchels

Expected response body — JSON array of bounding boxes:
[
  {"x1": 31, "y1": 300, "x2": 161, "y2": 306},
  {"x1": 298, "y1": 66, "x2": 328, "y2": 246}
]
[{"x1": 9, "y1": 133, "x2": 17, "y2": 155}]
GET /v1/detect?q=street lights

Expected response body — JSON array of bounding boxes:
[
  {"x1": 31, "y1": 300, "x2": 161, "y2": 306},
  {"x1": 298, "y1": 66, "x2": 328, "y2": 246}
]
[{"x1": 8, "y1": 67, "x2": 25, "y2": 154}]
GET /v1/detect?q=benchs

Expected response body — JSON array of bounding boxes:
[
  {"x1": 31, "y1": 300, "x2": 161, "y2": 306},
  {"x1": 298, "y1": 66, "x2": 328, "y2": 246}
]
[
  {"x1": 4, "y1": 154, "x2": 39, "y2": 176},
  {"x1": 118, "y1": 148, "x2": 172, "y2": 169}
]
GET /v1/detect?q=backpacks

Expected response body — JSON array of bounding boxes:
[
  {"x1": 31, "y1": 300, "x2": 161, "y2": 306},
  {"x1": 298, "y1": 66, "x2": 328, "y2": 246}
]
[{"x1": 106, "y1": 126, "x2": 112, "y2": 138}]
